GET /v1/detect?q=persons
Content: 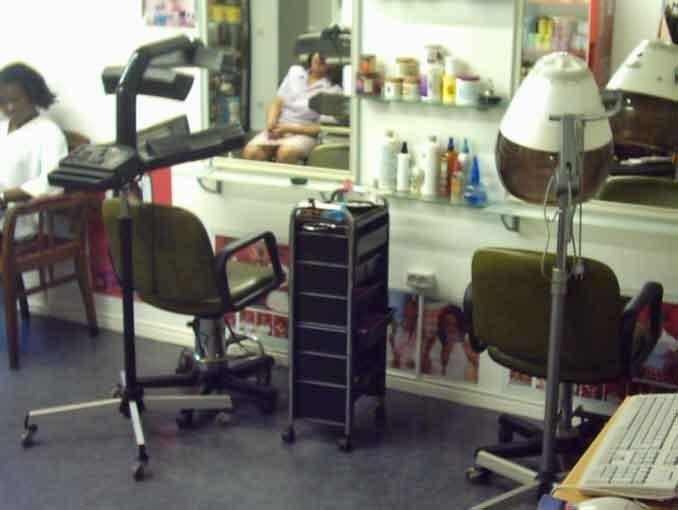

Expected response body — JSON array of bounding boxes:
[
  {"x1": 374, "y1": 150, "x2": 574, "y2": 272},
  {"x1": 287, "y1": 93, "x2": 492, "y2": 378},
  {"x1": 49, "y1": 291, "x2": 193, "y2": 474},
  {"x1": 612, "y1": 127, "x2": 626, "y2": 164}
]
[
  {"x1": 256, "y1": 314, "x2": 271, "y2": 336},
  {"x1": 0, "y1": 63, "x2": 69, "y2": 245},
  {"x1": 238, "y1": 311, "x2": 253, "y2": 332},
  {"x1": 385, "y1": 297, "x2": 478, "y2": 385},
  {"x1": 241, "y1": 51, "x2": 341, "y2": 164},
  {"x1": 273, "y1": 319, "x2": 287, "y2": 338}
]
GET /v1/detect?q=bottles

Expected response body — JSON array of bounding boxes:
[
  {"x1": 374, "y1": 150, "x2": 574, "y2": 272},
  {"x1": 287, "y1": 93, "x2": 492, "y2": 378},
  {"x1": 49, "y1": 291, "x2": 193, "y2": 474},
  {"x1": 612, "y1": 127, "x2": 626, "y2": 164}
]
[{"x1": 378, "y1": 130, "x2": 470, "y2": 195}]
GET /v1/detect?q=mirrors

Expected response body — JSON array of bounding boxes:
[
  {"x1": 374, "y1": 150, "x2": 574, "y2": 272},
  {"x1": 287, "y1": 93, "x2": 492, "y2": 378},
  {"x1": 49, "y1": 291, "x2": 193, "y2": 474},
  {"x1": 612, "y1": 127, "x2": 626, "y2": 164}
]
[
  {"x1": 194, "y1": 0, "x2": 361, "y2": 186},
  {"x1": 512, "y1": 1, "x2": 678, "y2": 222}
]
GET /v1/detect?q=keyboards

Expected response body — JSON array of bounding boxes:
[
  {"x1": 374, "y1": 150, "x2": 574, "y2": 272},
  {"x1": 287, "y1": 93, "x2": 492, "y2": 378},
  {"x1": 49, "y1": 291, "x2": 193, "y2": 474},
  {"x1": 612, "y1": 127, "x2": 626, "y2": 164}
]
[{"x1": 576, "y1": 391, "x2": 678, "y2": 503}]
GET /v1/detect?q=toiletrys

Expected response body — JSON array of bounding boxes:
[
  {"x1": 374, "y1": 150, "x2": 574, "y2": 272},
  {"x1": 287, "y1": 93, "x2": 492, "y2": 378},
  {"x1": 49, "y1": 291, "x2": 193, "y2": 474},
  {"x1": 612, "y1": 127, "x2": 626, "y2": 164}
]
[{"x1": 378, "y1": 128, "x2": 487, "y2": 206}]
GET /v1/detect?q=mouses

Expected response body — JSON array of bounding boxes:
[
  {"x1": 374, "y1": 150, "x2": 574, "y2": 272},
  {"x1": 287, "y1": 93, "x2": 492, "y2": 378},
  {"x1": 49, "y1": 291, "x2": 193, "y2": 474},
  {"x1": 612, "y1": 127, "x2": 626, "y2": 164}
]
[{"x1": 577, "y1": 496, "x2": 648, "y2": 510}]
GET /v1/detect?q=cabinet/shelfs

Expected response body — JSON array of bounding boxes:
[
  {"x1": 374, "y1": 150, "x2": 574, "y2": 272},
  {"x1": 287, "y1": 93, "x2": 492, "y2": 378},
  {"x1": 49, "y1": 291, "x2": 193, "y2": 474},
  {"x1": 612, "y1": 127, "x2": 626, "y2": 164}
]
[{"x1": 360, "y1": 89, "x2": 511, "y2": 213}]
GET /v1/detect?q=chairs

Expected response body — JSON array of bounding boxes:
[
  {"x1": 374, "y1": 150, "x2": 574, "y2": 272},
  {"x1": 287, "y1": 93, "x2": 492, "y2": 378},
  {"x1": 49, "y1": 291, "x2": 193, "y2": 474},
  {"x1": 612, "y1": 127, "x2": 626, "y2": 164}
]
[
  {"x1": 102, "y1": 198, "x2": 286, "y2": 430},
  {"x1": 463, "y1": 245, "x2": 664, "y2": 484},
  {"x1": 0, "y1": 133, "x2": 100, "y2": 369}
]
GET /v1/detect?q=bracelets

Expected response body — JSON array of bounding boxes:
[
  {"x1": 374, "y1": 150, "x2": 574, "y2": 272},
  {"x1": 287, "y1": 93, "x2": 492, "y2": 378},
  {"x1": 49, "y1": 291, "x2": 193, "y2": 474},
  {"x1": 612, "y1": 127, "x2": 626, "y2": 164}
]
[{"x1": 0, "y1": 192, "x2": 7, "y2": 208}]
